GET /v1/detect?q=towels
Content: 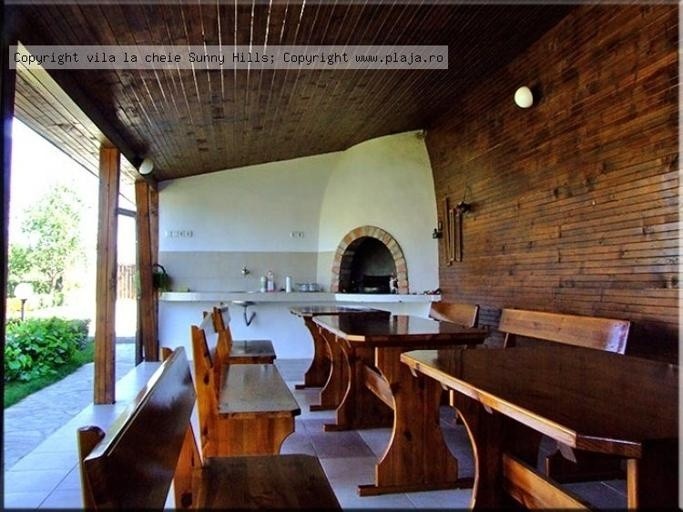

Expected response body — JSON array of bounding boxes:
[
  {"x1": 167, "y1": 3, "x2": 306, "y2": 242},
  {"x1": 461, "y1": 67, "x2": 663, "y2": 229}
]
[{"x1": 285, "y1": 276, "x2": 292, "y2": 293}]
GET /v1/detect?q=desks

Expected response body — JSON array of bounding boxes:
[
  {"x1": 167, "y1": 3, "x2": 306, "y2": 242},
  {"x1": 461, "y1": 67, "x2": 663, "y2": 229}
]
[
  {"x1": 287, "y1": 305, "x2": 391, "y2": 411},
  {"x1": 399, "y1": 340, "x2": 678, "y2": 512},
  {"x1": 311, "y1": 314, "x2": 491, "y2": 496}
]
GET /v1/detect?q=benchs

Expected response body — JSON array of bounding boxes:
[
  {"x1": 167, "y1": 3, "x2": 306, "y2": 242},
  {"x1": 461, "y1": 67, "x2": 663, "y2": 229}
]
[
  {"x1": 496, "y1": 304, "x2": 631, "y2": 482},
  {"x1": 428, "y1": 300, "x2": 480, "y2": 426},
  {"x1": 214, "y1": 305, "x2": 276, "y2": 365},
  {"x1": 191, "y1": 311, "x2": 301, "y2": 456},
  {"x1": 78, "y1": 347, "x2": 343, "y2": 510}
]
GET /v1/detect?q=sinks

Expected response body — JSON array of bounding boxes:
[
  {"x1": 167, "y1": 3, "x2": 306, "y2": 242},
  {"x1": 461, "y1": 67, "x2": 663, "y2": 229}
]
[{"x1": 226, "y1": 290, "x2": 258, "y2": 293}]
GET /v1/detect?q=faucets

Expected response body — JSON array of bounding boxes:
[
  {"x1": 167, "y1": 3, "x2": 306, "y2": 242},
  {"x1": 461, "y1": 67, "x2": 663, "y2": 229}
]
[{"x1": 240, "y1": 265, "x2": 250, "y2": 275}]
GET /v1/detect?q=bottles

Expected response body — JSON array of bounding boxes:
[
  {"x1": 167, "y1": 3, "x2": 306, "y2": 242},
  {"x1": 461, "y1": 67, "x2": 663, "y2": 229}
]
[{"x1": 260, "y1": 270, "x2": 274, "y2": 293}]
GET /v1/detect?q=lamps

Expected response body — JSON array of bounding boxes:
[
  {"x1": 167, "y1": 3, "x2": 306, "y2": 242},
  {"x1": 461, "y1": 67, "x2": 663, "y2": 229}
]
[
  {"x1": 138, "y1": 157, "x2": 154, "y2": 175},
  {"x1": 513, "y1": 85, "x2": 543, "y2": 108}
]
